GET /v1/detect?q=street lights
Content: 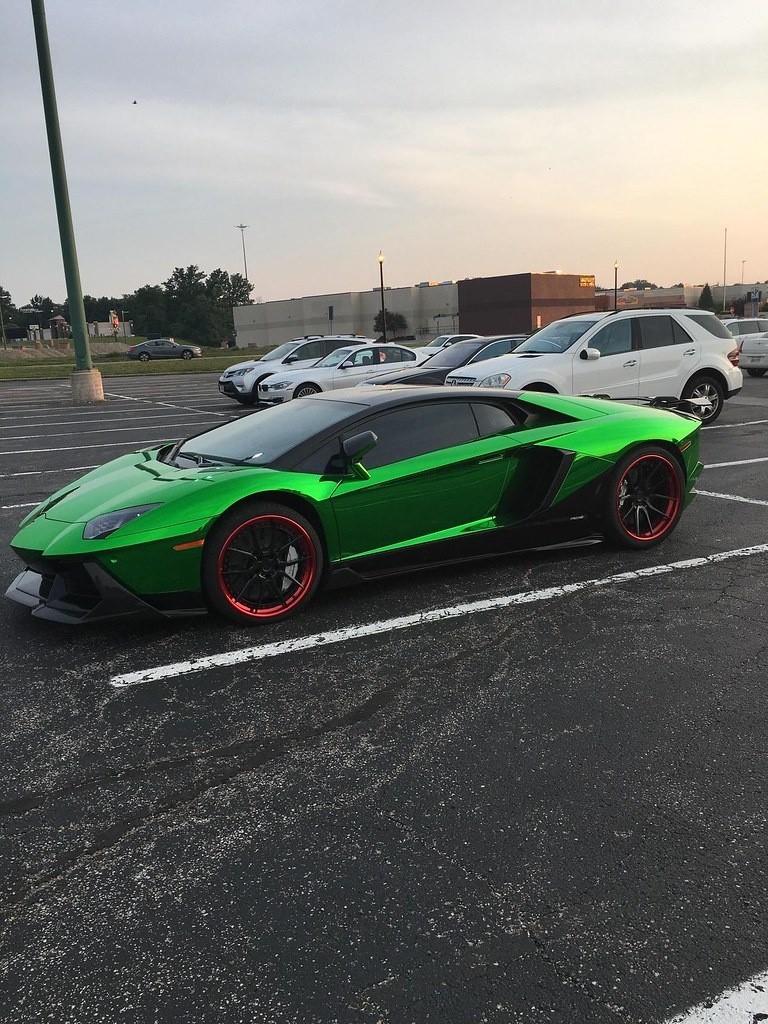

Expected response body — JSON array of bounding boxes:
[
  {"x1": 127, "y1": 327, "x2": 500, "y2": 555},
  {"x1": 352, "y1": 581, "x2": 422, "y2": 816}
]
[
  {"x1": 613, "y1": 260, "x2": 619, "y2": 311},
  {"x1": 377, "y1": 249, "x2": 387, "y2": 343},
  {"x1": 236, "y1": 223, "x2": 250, "y2": 285}
]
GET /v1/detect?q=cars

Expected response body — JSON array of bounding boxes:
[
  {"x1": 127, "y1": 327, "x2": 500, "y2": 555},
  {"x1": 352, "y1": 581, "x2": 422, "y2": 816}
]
[
  {"x1": 126, "y1": 338, "x2": 202, "y2": 362},
  {"x1": 256, "y1": 342, "x2": 432, "y2": 407},
  {"x1": 719, "y1": 318, "x2": 768, "y2": 378},
  {"x1": 412, "y1": 333, "x2": 484, "y2": 357},
  {"x1": 354, "y1": 333, "x2": 561, "y2": 387}
]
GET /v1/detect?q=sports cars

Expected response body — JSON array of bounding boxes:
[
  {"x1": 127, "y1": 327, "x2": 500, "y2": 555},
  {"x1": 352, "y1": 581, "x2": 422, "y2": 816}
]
[{"x1": 4, "y1": 384, "x2": 705, "y2": 635}]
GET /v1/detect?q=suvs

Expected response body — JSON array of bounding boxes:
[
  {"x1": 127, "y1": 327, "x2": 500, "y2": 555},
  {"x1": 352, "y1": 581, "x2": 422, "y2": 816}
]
[
  {"x1": 218, "y1": 334, "x2": 387, "y2": 407},
  {"x1": 443, "y1": 305, "x2": 744, "y2": 426}
]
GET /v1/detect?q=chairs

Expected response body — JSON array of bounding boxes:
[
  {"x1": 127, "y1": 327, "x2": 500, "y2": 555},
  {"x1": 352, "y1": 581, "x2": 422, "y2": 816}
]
[
  {"x1": 300, "y1": 346, "x2": 311, "y2": 360},
  {"x1": 359, "y1": 433, "x2": 394, "y2": 470},
  {"x1": 362, "y1": 356, "x2": 373, "y2": 363}
]
